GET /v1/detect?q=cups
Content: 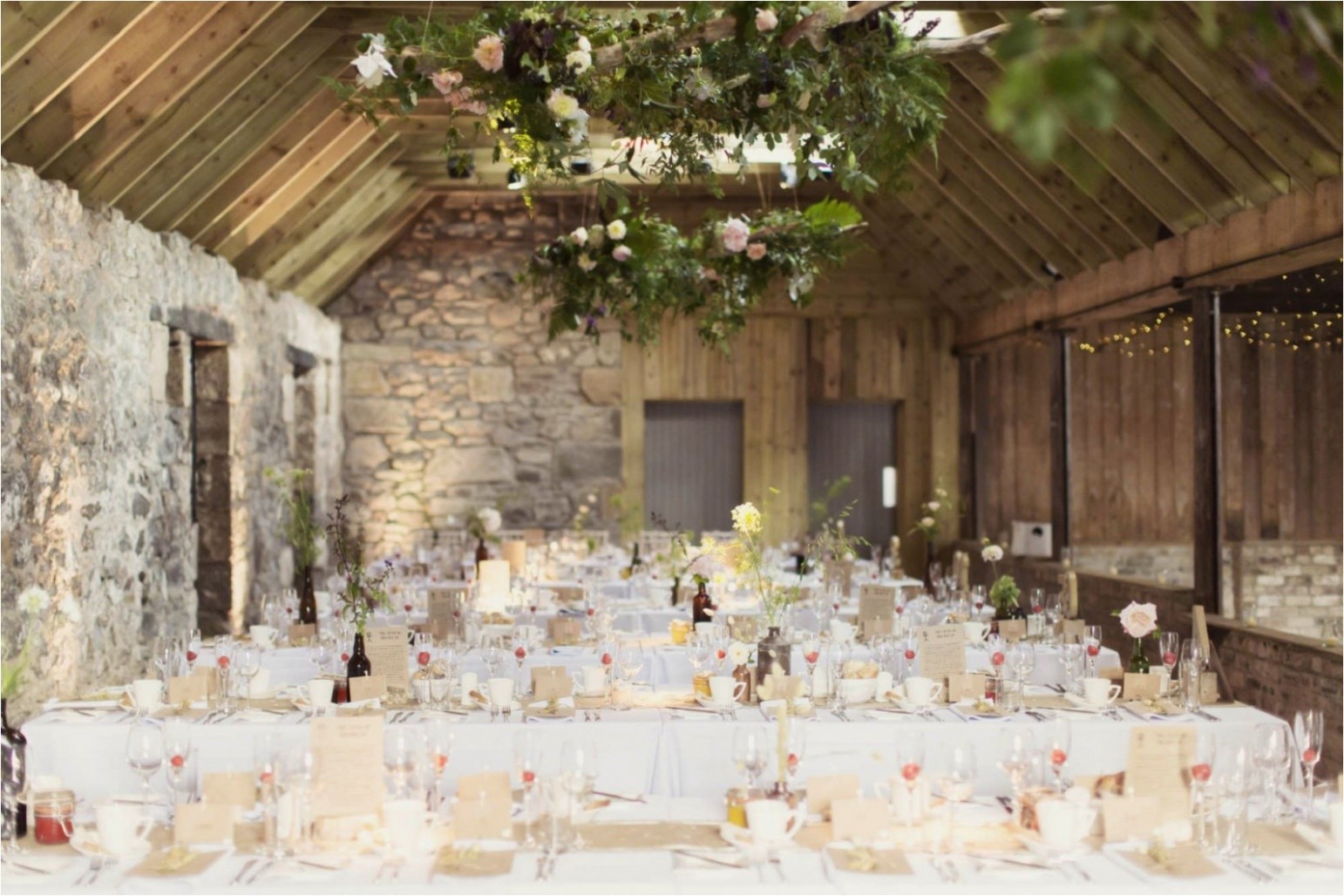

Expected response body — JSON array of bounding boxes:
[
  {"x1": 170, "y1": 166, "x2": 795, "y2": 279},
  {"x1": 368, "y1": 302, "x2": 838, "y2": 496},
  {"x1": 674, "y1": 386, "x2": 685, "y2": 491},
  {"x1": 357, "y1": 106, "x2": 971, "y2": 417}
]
[{"x1": 33, "y1": 790, "x2": 75, "y2": 845}]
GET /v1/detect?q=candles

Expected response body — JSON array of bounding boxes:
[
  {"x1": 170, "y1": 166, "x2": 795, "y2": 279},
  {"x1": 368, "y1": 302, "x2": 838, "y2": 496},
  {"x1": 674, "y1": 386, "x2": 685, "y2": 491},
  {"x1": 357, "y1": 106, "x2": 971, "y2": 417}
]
[{"x1": 881, "y1": 465, "x2": 898, "y2": 507}]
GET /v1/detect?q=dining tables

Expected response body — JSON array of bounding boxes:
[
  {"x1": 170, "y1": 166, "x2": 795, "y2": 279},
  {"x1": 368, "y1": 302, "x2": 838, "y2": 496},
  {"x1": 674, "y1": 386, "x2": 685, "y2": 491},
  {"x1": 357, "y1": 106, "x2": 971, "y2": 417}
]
[{"x1": 1, "y1": 526, "x2": 1343, "y2": 895}]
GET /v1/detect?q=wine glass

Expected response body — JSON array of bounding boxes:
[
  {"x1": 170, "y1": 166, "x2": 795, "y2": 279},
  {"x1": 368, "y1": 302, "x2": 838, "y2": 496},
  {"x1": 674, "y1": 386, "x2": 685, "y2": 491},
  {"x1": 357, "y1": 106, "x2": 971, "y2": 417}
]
[
  {"x1": 1252, "y1": 719, "x2": 1291, "y2": 826},
  {"x1": 126, "y1": 590, "x2": 1245, "y2": 888},
  {"x1": 1294, "y1": 708, "x2": 1329, "y2": 812}
]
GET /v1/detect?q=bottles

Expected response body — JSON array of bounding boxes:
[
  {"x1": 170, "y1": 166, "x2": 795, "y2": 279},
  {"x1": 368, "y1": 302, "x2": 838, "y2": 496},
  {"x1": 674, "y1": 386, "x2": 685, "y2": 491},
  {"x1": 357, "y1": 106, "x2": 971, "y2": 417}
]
[
  {"x1": 694, "y1": 583, "x2": 712, "y2": 623},
  {"x1": 475, "y1": 539, "x2": 487, "y2": 565},
  {"x1": 0, "y1": 695, "x2": 29, "y2": 837},
  {"x1": 300, "y1": 568, "x2": 317, "y2": 624}
]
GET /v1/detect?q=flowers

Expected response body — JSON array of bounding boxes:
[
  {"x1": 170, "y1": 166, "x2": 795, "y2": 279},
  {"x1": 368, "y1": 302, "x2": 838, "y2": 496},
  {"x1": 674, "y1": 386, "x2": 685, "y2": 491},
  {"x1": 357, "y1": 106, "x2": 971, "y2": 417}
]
[
  {"x1": 464, "y1": 507, "x2": 504, "y2": 542},
  {"x1": 321, "y1": 0, "x2": 955, "y2": 360},
  {"x1": 262, "y1": 465, "x2": 326, "y2": 565},
  {"x1": 912, "y1": 475, "x2": 955, "y2": 540},
  {"x1": 698, "y1": 495, "x2": 875, "y2": 626},
  {"x1": 322, "y1": 493, "x2": 395, "y2": 633}
]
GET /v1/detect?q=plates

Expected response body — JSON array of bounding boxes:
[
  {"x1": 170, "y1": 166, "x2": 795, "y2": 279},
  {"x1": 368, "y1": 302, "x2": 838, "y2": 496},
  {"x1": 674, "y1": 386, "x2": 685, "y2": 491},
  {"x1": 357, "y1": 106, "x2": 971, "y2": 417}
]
[{"x1": 71, "y1": 829, "x2": 150, "y2": 859}]
